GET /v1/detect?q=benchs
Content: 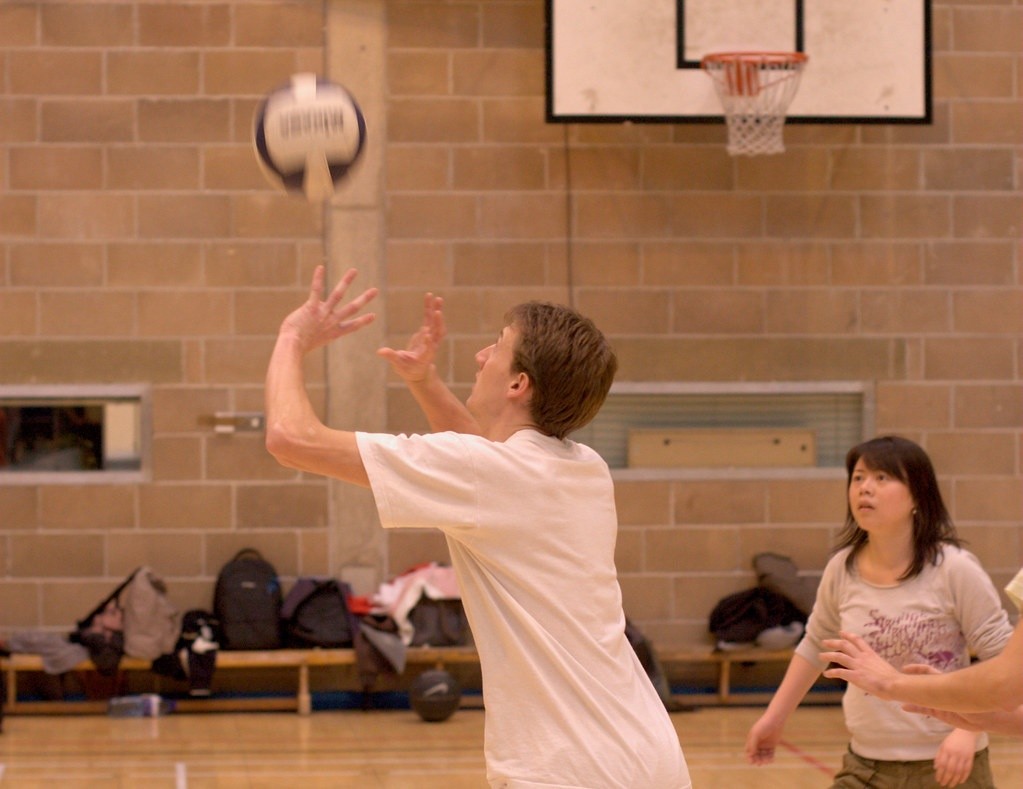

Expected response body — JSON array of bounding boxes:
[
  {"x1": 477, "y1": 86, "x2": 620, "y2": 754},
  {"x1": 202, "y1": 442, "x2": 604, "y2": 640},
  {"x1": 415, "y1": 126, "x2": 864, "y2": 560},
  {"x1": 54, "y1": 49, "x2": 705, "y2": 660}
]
[{"x1": 0, "y1": 645, "x2": 846, "y2": 715}]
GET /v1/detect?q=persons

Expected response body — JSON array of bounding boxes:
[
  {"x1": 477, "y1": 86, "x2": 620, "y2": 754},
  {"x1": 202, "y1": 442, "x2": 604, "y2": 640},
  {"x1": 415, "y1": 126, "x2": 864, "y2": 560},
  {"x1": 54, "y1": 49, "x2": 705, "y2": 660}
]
[
  {"x1": 264, "y1": 263, "x2": 690, "y2": 789},
  {"x1": 745, "y1": 434, "x2": 1023, "y2": 789}
]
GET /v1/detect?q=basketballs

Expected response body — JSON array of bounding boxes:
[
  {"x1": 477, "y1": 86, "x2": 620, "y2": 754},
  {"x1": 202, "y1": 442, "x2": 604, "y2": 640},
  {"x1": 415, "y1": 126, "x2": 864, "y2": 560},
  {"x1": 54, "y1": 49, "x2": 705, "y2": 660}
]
[
  {"x1": 410, "y1": 670, "x2": 460, "y2": 721},
  {"x1": 252, "y1": 73, "x2": 368, "y2": 205}
]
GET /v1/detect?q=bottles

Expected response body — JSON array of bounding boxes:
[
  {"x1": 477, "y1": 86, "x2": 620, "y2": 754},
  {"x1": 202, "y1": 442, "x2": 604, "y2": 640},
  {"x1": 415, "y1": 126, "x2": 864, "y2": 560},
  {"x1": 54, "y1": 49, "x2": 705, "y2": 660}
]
[{"x1": 109, "y1": 693, "x2": 178, "y2": 717}]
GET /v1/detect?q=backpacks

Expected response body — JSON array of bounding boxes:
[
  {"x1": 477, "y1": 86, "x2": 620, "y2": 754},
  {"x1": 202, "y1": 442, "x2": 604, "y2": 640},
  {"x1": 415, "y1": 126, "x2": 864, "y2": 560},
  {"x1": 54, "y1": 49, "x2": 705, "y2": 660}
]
[
  {"x1": 284, "y1": 573, "x2": 358, "y2": 650},
  {"x1": 213, "y1": 549, "x2": 282, "y2": 649}
]
